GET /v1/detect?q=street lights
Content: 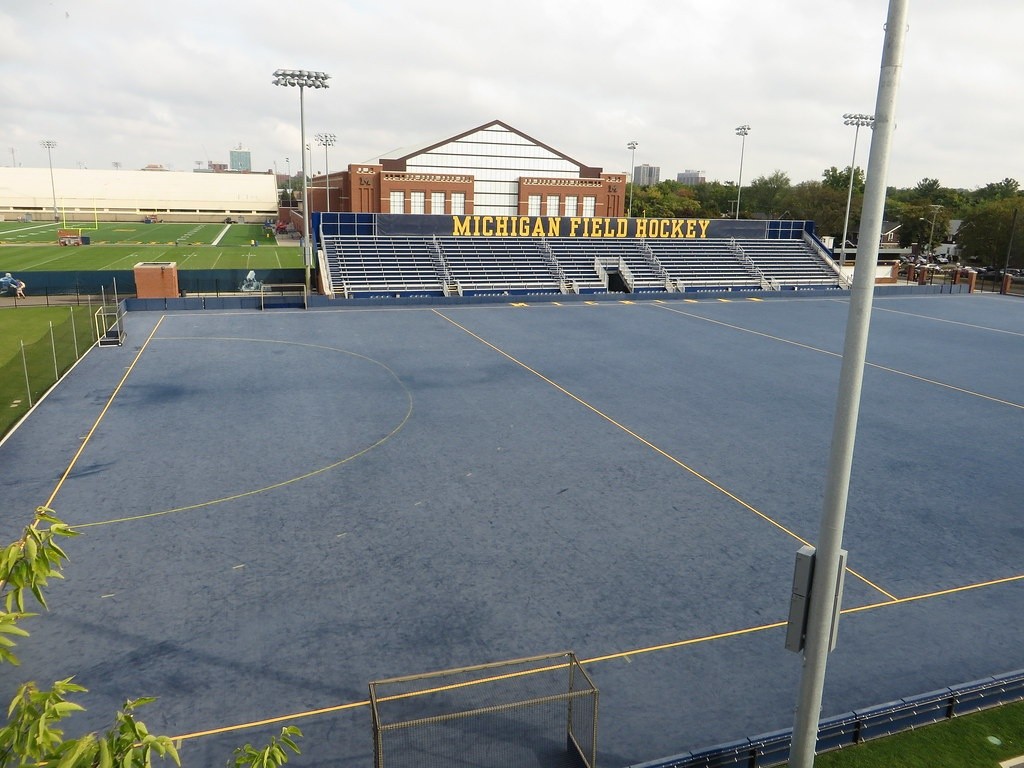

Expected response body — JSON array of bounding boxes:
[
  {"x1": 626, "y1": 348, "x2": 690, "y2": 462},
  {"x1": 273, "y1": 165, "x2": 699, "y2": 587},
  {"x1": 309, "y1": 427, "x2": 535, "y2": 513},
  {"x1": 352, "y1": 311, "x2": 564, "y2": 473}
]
[
  {"x1": 626, "y1": 140, "x2": 638, "y2": 218},
  {"x1": 272, "y1": 67, "x2": 331, "y2": 295},
  {"x1": 919, "y1": 217, "x2": 934, "y2": 266},
  {"x1": 40, "y1": 139, "x2": 60, "y2": 225},
  {"x1": 839, "y1": 113, "x2": 875, "y2": 267},
  {"x1": 315, "y1": 132, "x2": 337, "y2": 212},
  {"x1": 734, "y1": 124, "x2": 751, "y2": 221}
]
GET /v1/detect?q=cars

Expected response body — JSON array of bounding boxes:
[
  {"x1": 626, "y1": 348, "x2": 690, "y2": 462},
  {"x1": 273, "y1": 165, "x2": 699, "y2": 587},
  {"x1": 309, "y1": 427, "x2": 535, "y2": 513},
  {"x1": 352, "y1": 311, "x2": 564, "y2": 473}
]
[{"x1": 900, "y1": 255, "x2": 1024, "y2": 279}]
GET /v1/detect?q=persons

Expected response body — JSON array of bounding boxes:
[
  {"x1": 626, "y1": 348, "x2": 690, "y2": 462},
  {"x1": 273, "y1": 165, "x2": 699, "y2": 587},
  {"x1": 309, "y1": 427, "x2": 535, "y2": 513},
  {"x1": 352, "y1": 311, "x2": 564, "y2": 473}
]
[
  {"x1": 272, "y1": 227, "x2": 277, "y2": 236},
  {"x1": 16, "y1": 279, "x2": 25, "y2": 299},
  {"x1": 250, "y1": 240, "x2": 258, "y2": 246},
  {"x1": 0, "y1": 273, "x2": 17, "y2": 295}
]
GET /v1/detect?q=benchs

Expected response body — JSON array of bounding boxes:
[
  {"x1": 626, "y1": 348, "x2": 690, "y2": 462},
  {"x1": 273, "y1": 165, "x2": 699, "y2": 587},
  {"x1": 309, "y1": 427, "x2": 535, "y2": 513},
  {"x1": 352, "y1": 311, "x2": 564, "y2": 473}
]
[{"x1": 323, "y1": 235, "x2": 834, "y2": 292}]
